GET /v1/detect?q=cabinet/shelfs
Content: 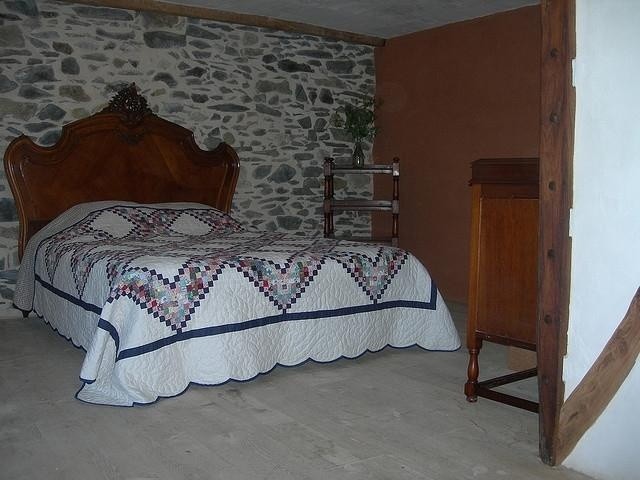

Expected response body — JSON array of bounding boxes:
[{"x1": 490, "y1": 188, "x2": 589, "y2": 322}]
[
  {"x1": 322, "y1": 156, "x2": 401, "y2": 248},
  {"x1": 465, "y1": 156, "x2": 541, "y2": 416}
]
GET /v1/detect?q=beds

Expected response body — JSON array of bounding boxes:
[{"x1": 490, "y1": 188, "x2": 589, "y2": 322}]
[{"x1": 5, "y1": 80, "x2": 461, "y2": 407}]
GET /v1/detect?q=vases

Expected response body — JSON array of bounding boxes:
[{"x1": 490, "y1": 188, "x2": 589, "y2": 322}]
[{"x1": 352, "y1": 141, "x2": 365, "y2": 169}]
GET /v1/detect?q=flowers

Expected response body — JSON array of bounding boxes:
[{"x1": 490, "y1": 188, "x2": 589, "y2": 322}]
[{"x1": 331, "y1": 91, "x2": 384, "y2": 142}]
